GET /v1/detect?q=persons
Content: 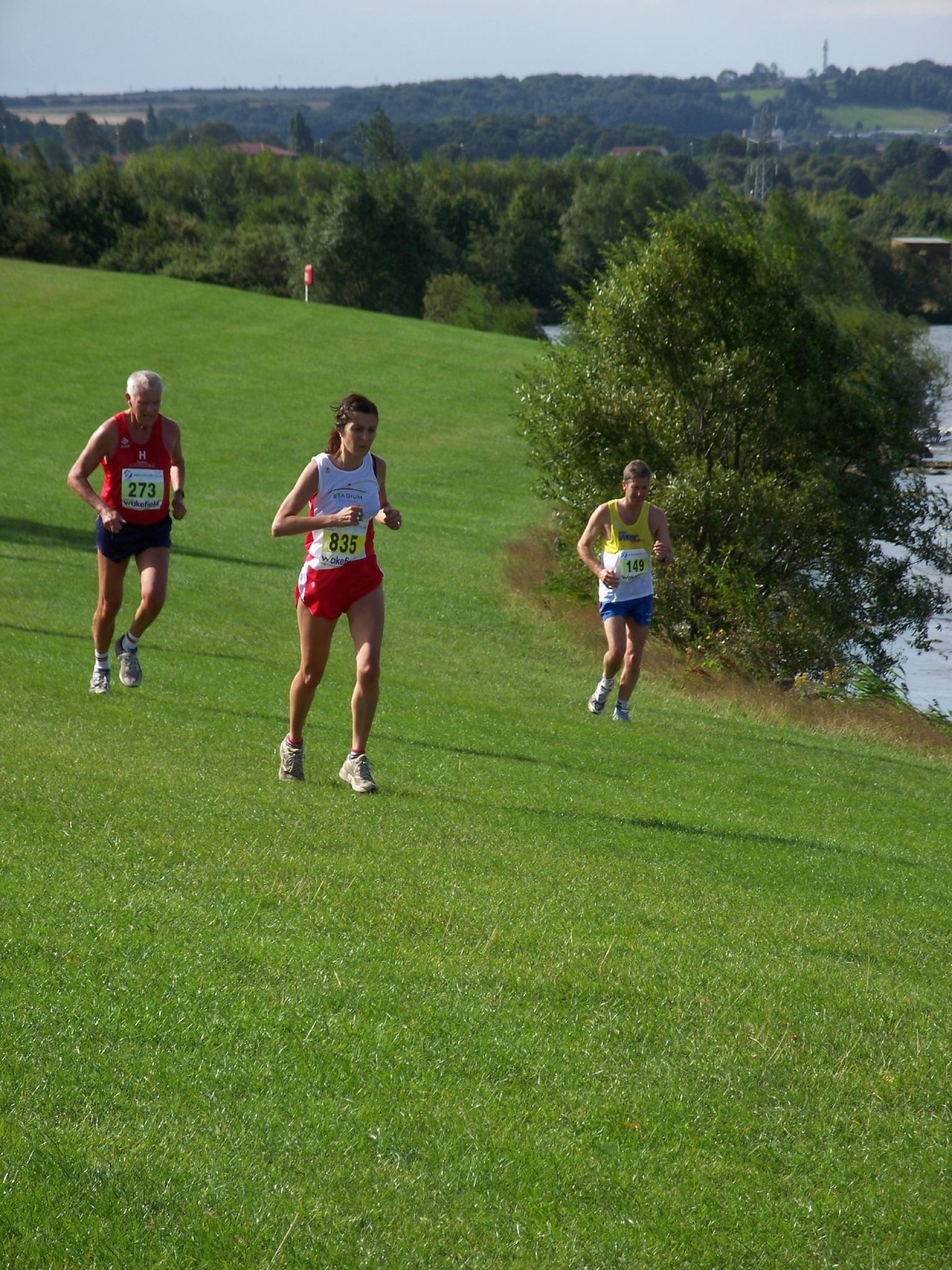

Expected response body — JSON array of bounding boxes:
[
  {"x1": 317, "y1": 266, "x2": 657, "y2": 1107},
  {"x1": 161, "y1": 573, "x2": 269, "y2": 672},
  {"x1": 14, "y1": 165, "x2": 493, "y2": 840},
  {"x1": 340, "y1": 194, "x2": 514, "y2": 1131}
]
[
  {"x1": 67, "y1": 371, "x2": 187, "y2": 694},
  {"x1": 272, "y1": 393, "x2": 402, "y2": 795},
  {"x1": 577, "y1": 459, "x2": 673, "y2": 724}
]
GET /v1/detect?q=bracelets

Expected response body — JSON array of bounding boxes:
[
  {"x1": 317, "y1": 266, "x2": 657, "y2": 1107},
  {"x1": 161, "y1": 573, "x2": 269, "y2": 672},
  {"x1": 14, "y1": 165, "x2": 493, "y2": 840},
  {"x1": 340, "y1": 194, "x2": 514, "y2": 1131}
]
[{"x1": 173, "y1": 489, "x2": 185, "y2": 498}]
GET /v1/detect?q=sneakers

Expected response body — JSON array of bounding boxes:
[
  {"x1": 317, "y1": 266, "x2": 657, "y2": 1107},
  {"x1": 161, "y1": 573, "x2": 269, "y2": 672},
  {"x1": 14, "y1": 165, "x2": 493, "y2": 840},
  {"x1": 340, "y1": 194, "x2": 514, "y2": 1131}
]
[
  {"x1": 278, "y1": 732, "x2": 305, "y2": 782},
  {"x1": 339, "y1": 754, "x2": 379, "y2": 794},
  {"x1": 588, "y1": 679, "x2": 614, "y2": 715},
  {"x1": 89, "y1": 665, "x2": 111, "y2": 694},
  {"x1": 115, "y1": 634, "x2": 142, "y2": 687},
  {"x1": 613, "y1": 707, "x2": 629, "y2": 721}
]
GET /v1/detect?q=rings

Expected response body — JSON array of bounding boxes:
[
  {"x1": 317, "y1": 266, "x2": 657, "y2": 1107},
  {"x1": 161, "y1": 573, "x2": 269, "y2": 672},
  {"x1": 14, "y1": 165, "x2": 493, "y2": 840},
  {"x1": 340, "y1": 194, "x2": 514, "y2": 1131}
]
[
  {"x1": 358, "y1": 511, "x2": 361, "y2": 516},
  {"x1": 397, "y1": 521, "x2": 400, "y2": 525},
  {"x1": 357, "y1": 517, "x2": 360, "y2": 522}
]
